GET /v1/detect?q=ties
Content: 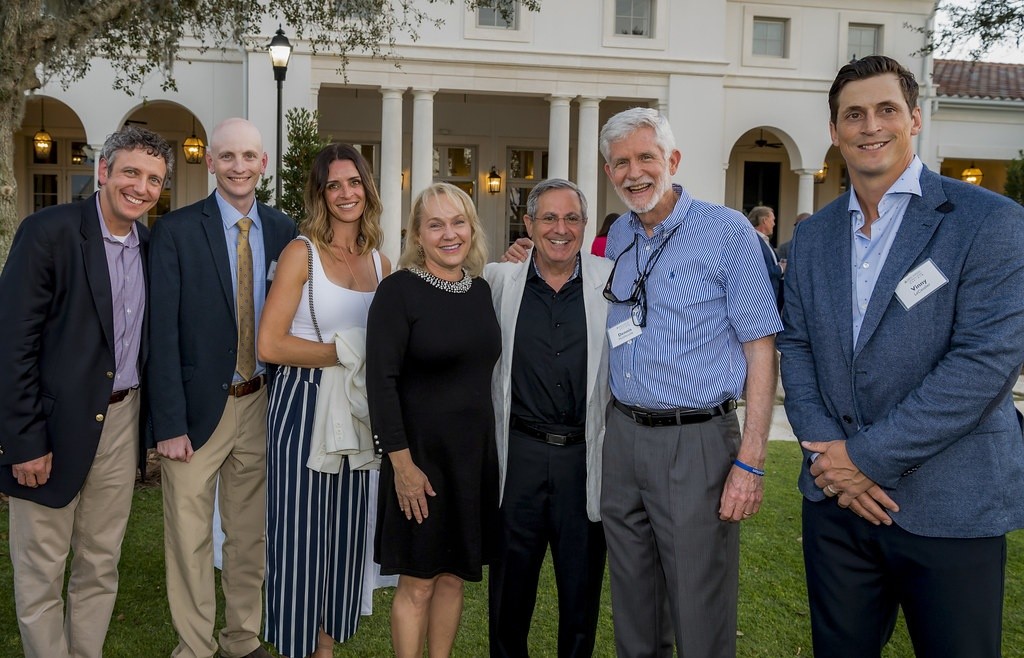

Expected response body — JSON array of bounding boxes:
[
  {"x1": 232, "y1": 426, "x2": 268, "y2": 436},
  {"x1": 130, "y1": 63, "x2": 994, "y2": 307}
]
[{"x1": 236, "y1": 217, "x2": 257, "y2": 381}]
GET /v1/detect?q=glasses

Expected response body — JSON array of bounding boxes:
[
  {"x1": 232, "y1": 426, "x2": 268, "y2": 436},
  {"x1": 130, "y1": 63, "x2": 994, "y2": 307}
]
[
  {"x1": 603, "y1": 242, "x2": 658, "y2": 306},
  {"x1": 630, "y1": 278, "x2": 647, "y2": 330},
  {"x1": 533, "y1": 215, "x2": 586, "y2": 225}
]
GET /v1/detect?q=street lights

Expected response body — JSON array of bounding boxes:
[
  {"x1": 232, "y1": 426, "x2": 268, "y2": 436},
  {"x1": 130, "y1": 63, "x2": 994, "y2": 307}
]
[{"x1": 266, "y1": 24, "x2": 293, "y2": 212}]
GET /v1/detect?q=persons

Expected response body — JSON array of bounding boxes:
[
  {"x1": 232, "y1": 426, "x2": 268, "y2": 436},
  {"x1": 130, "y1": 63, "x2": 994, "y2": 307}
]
[
  {"x1": 258, "y1": 143, "x2": 391, "y2": 658},
  {"x1": 779, "y1": 212, "x2": 813, "y2": 263},
  {"x1": 146, "y1": 117, "x2": 300, "y2": 658},
  {"x1": 0, "y1": 123, "x2": 176, "y2": 658},
  {"x1": 779, "y1": 55, "x2": 1024, "y2": 658},
  {"x1": 364, "y1": 108, "x2": 785, "y2": 658}
]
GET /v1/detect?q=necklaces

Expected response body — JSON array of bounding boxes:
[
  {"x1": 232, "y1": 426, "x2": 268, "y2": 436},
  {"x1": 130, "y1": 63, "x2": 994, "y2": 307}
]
[{"x1": 338, "y1": 242, "x2": 375, "y2": 311}]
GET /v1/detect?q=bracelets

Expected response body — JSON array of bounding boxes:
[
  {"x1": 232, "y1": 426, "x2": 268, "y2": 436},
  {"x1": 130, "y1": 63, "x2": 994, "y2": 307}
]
[
  {"x1": 734, "y1": 458, "x2": 765, "y2": 476},
  {"x1": 337, "y1": 356, "x2": 341, "y2": 365}
]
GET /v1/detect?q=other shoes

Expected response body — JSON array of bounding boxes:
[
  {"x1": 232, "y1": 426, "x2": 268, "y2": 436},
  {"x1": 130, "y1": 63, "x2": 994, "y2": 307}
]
[{"x1": 219, "y1": 643, "x2": 273, "y2": 658}]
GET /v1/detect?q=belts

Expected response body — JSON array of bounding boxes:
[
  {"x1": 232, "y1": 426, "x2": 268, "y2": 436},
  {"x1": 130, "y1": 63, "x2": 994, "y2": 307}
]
[
  {"x1": 228, "y1": 375, "x2": 268, "y2": 398},
  {"x1": 613, "y1": 397, "x2": 734, "y2": 427},
  {"x1": 508, "y1": 422, "x2": 588, "y2": 446},
  {"x1": 109, "y1": 389, "x2": 129, "y2": 404}
]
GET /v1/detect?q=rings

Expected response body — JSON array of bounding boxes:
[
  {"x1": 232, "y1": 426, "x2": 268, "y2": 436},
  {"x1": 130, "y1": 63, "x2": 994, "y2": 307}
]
[
  {"x1": 827, "y1": 486, "x2": 839, "y2": 495},
  {"x1": 744, "y1": 512, "x2": 752, "y2": 515}
]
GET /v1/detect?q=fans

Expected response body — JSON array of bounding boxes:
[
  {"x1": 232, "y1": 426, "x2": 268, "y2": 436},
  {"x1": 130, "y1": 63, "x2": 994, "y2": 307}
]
[
  {"x1": 735, "y1": 129, "x2": 783, "y2": 150},
  {"x1": 124, "y1": 117, "x2": 146, "y2": 126}
]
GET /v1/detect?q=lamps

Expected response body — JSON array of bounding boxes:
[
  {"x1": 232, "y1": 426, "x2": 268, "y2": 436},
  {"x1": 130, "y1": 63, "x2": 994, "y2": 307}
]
[
  {"x1": 35, "y1": 95, "x2": 51, "y2": 159},
  {"x1": 962, "y1": 160, "x2": 984, "y2": 186},
  {"x1": 183, "y1": 115, "x2": 204, "y2": 164},
  {"x1": 814, "y1": 162, "x2": 828, "y2": 183},
  {"x1": 487, "y1": 165, "x2": 502, "y2": 195}
]
